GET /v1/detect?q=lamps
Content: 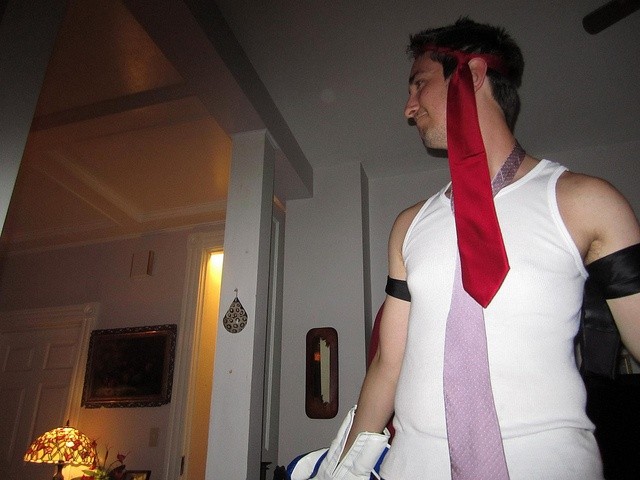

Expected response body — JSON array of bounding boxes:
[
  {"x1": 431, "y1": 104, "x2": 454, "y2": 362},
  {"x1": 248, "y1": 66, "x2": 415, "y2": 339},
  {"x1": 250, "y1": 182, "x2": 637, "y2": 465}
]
[{"x1": 22, "y1": 420, "x2": 93, "y2": 480}]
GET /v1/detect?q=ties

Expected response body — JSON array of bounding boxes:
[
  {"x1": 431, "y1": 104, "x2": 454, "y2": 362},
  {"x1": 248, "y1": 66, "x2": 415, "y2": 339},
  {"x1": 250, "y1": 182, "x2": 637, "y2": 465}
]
[
  {"x1": 421, "y1": 43, "x2": 510, "y2": 308},
  {"x1": 442, "y1": 141, "x2": 527, "y2": 480}
]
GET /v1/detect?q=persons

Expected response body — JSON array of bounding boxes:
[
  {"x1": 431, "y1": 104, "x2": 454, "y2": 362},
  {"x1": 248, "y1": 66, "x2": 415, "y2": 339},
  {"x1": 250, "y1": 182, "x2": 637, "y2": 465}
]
[{"x1": 312, "y1": 19, "x2": 638, "y2": 478}]
